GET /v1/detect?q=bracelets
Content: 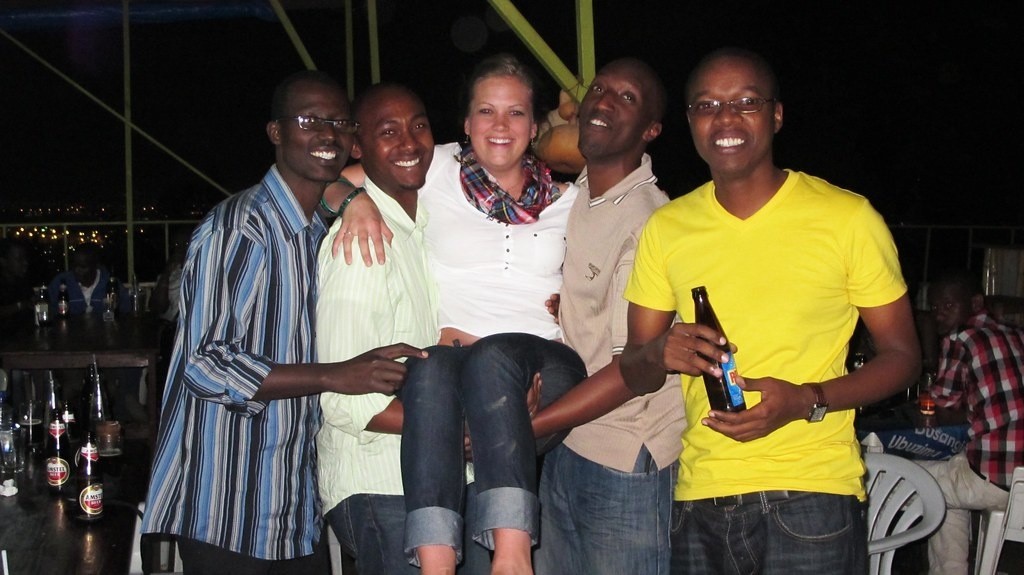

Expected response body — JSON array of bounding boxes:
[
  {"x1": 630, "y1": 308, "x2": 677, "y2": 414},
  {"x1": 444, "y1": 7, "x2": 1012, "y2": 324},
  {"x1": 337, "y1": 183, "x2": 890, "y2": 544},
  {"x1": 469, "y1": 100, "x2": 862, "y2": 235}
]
[
  {"x1": 319, "y1": 177, "x2": 355, "y2": 216},
  {"x1": 337, "y1": 186, "x2": 364, "y2": 217}
]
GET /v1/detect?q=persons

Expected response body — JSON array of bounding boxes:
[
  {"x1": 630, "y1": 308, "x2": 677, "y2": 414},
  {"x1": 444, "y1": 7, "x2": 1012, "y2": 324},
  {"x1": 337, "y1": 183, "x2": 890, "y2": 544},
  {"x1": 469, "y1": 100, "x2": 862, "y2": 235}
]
[
  {"x1": 620, "y1": 45, "x2": 922, "y2": 575},
  {"x1": 865, "y1": 255, "x2": 1024, "y2": 575},
  {"x1": 0, "y1": 226, "x2": 191, "y2": 454},
  {"x1": 140, "y1": 70, "x2": 429, "y2": 575},
  {"x1": 315, "y1": 49, "x2": 686, "y2": 575}
]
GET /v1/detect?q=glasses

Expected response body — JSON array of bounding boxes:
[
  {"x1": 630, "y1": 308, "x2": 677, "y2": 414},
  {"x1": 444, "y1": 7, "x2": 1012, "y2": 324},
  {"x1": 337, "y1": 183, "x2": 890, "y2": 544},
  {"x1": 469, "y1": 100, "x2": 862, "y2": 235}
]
[
  {"x1": 276, "y1": 116, "x2": 361, "y2": 134},
  {"x1": 688, "y1": 97, "x2": 776, "y2": 113}
]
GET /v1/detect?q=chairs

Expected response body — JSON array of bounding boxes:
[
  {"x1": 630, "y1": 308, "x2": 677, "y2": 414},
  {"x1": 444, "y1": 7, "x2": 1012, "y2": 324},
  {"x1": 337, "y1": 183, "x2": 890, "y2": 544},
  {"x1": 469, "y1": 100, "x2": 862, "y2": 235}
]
[
  {"x1": 862, "y1": 453, "x2": 946, "y2": 575},
  {"x1": 973, "y1": 467, "x2": 1024, "y2": 575}
]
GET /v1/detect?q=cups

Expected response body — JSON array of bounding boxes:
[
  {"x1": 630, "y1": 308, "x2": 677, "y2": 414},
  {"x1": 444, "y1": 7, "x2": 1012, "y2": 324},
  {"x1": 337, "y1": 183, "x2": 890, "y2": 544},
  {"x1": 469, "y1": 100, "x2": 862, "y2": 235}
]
[
  {"x1": 33, "y1": 286, "x2": 41, "y2": 296},
  {"x1": 93, "y1": 420, "x2": 123, "y2": 457},
  {"x1": 19, "y1": 400, "x2": 44, "y2": 456},
  {"x1": 101, "y1": 298, "x2": 115, "y2": 322},
  {"x1": 919, "y1": 375, "x2": 936, "y2": 415},
  {"x1": 0, "y1": 424, "x2": 22, "y2": 497}
]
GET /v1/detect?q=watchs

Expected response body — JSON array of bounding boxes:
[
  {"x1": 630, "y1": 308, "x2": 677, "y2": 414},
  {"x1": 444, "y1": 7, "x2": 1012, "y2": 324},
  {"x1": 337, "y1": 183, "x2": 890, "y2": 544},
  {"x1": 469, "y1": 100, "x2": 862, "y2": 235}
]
[{"x1": 803, "y1": 383, "x2": 829, "y2": 422}]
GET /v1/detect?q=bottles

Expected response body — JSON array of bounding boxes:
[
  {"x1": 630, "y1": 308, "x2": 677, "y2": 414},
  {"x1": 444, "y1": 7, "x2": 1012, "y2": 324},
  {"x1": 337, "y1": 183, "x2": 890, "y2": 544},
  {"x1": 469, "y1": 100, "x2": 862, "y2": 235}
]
[
  {"x1": 691, "y1": 286, "x2": 747, "y2": 413},
  {"x1": 34, "y1": 280, "x2": 48, "y2": 326},
  {"x1": 57, "y1": 279, "x2": 70, "y2": 319},
  {"x1": 106, "y1": 264, "x2": 120, "y2": 318},
  {"x1": 129, "y1": 273, "x2": 145, "y2": 319},
  {"x1": 44, "y1": 361, "x2": 113, "y2": 523},
  {"x1": 0, "y1": 368, "x2": 13, "y2": 423},
  {"x1": 852, "y1": 352, "x2": 867, "y2": 416}
]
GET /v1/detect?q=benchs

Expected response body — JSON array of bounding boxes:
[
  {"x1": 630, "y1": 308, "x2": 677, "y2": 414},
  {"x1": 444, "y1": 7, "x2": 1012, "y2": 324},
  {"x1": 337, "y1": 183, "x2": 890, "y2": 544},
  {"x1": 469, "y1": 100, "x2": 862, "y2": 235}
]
[{"x1": 0, "y1": 312, "x2": 176, "y2": 474}]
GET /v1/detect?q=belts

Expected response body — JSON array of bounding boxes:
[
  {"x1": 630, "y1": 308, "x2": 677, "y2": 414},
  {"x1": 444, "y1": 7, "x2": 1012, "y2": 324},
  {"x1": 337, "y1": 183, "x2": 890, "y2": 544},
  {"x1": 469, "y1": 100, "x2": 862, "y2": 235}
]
[{"x1": 706, "y1": 491, "x2": 817, "y2": 505}]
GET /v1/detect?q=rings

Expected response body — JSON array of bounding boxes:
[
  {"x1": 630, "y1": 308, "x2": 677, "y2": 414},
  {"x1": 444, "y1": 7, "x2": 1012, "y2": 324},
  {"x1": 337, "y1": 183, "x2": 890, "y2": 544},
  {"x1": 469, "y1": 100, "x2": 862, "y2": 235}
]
[
  {"x1": 529, "y1": 412, "x2": 534, "y2": 417},
  {"x1": 344, "y1": 231, "x2": 353, "y2": 236}
]
